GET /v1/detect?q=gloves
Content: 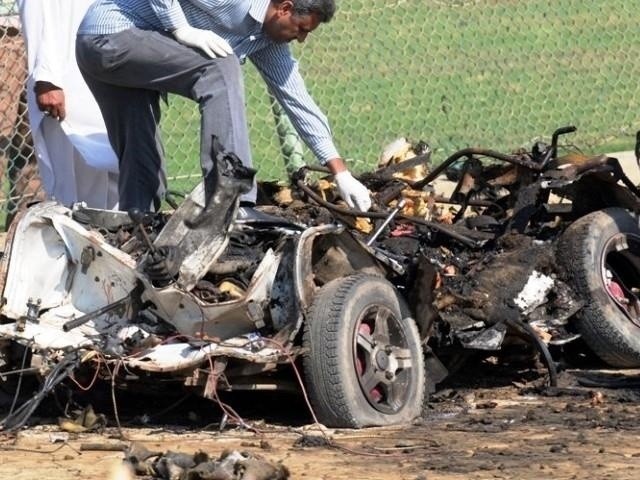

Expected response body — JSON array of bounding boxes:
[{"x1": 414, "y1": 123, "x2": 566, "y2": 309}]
[
  {"x1": 171, "y1": 24, "x2": 236, "y2": 61},
  {"x1": 332, "y1": 169, "x2": 373, "y2": 214}
]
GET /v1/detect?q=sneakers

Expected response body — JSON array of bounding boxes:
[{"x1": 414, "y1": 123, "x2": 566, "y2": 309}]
[{"x1": 234, "y1": 201, "x2": 290, "y2": 228}]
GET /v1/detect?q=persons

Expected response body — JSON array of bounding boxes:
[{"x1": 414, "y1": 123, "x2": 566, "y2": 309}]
[
  {"x1": 15, "y1": 0, "x2": 121, "y2": 213},
  {"x1": 76, "y1": 1, "x2": 373, "y2": 222}
]
